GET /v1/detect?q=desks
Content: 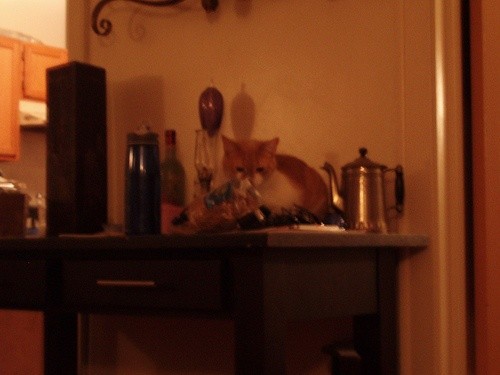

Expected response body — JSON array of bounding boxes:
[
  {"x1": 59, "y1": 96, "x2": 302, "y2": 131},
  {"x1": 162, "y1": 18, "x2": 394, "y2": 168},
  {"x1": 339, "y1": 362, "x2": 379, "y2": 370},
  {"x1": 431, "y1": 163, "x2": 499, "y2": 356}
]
[{"x1": 0, "y1": 225, "x2": 430, "y2": 375}]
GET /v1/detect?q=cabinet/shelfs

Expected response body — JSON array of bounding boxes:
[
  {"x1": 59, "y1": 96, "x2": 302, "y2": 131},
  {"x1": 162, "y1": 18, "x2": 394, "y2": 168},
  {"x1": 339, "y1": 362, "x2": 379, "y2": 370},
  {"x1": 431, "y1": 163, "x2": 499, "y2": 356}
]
[
  {"x1": 0, "y1": 35, "x2": 25, "y2": 162},
  {"x1": 19, "y1": 43, "x2": 69, "y2": 130}
]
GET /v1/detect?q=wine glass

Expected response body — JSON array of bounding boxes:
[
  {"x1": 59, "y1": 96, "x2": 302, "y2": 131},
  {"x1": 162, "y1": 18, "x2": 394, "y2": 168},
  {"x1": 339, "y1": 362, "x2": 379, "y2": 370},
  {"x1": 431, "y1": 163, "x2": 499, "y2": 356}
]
[{"x1": 192, "y1": 126, "x2": 219, "y2": 193}]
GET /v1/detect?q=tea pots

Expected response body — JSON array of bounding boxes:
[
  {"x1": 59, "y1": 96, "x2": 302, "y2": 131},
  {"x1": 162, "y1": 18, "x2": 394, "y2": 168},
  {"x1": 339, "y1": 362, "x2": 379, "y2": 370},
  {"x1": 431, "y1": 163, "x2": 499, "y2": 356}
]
[{"x1": 322, "y1": 147, "x2": 406, "y2": 233}]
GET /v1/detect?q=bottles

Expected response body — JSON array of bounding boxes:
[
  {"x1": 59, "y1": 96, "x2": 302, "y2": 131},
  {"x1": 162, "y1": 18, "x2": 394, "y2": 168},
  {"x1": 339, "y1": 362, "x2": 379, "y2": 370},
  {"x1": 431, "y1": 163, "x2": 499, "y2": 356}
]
[
  {"x1": 122, "y1": 131, "x2": 163, "y2": 236},
  {"x1": 154, "y1": 127, "x2": 188, "y2": 235}
]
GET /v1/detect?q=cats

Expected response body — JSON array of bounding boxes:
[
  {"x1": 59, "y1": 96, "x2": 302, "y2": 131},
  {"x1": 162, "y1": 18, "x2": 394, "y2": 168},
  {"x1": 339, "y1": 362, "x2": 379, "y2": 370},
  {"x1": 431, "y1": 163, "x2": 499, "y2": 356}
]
[{"x1": 219, "y1": 133, "x2": 328, "y2": 221}]
